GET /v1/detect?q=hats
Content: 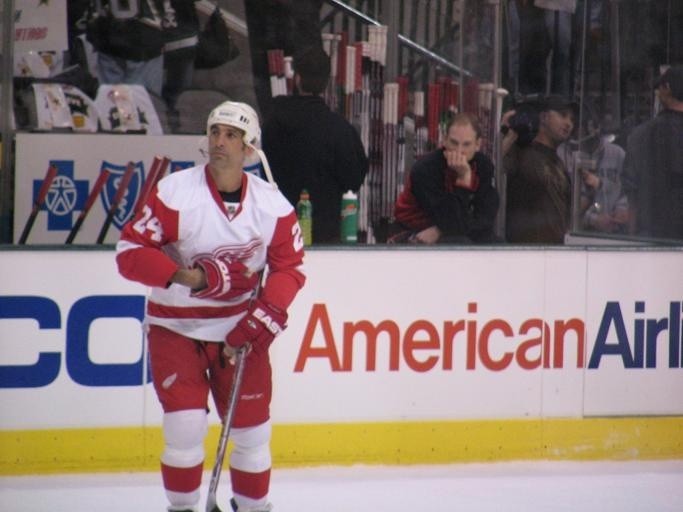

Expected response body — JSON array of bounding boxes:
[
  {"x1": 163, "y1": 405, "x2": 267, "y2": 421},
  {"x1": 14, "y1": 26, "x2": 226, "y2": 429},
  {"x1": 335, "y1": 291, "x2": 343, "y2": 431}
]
[
  {"x1": 652, "y1": 67, "x2": 682, "y2": 95},
  {"x1": 535, "y1": 94, "x2": 581, "y2": 116}
]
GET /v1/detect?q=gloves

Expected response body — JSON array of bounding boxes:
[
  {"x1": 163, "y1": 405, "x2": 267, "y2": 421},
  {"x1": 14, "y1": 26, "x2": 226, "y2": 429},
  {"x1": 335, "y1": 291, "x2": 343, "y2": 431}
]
[
  {"x1": 190, "y1": 256, "x2": 259, "y2": 302},
  {"x1": 224, "y1": 299, "x2": 287, "y2": 365}
]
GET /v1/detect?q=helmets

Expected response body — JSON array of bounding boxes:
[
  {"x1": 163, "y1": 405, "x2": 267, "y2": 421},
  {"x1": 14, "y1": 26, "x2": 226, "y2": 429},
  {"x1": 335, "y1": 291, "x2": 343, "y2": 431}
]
[{"x1": 207, "y1": 100, "x2": 262, "y2": 145}]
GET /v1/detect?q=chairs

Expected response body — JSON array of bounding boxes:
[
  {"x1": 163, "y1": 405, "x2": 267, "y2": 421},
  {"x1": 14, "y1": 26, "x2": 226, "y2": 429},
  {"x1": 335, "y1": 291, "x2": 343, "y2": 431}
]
[{"x1": 13, "y1": 77, "x2": 231, "y2": 135}]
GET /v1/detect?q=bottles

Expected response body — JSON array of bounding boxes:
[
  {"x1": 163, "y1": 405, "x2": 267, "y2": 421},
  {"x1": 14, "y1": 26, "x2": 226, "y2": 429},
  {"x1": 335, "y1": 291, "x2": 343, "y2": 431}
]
[
  {"x1": 294, "y1": 189, "x2": 312, "y2": 247},
  {"x1": 338, "y1": 188, "x2": 360, "y2": 245}
]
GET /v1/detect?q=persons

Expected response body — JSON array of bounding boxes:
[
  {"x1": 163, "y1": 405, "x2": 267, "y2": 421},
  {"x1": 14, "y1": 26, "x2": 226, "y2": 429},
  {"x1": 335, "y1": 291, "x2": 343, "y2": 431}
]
[
  {"x1": 260, "y1": 46, "x2": 369, "y2": 244},
  {"x1": 556, "y1": 116, "x2": 629, "y2": 236},
  {"x1": 66, "y1": 0, "x2": 242, "y2": 133},
  {"x1": 506, "y1": 95, "x2": 603, "y2": 245},
  {"x1": 394, "y1": 112, "x2": 500, "y2": 245},
  {"x1": 619, "y1": 64, "x2": 683, "y2": 240},
  {"x1": 115, "y1": 101, "x2": 307, "y2": 512}
]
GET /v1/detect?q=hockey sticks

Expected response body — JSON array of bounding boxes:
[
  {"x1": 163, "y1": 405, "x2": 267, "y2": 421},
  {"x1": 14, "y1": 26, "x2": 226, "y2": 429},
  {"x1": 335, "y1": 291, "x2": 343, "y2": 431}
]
[{"x1": 206, "y1": 346, "x2": 246, "y2": 512}]
